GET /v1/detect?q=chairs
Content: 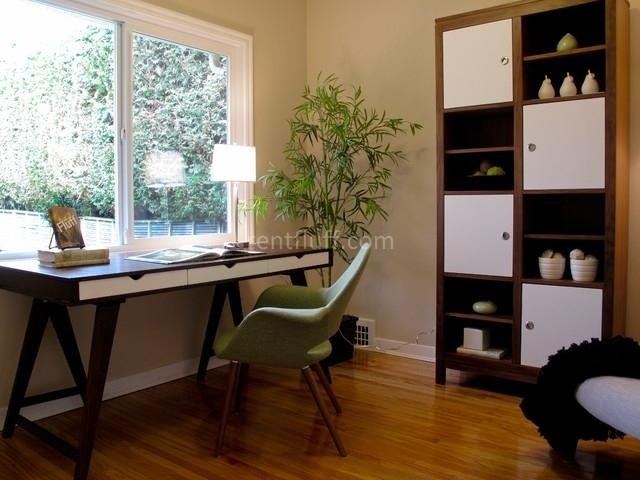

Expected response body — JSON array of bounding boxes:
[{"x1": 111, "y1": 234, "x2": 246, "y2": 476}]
[{"x1": 211, "y1": 240, "x2": 367, "y2": 458}]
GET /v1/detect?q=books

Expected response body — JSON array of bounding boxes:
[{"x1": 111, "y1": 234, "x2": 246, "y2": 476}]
[
  {"x1": 38, "y1": 247, "x2": 110, "y2": 268},
  {"x1": 126, "y1": 244, "x2": 266, "y2": 265}
]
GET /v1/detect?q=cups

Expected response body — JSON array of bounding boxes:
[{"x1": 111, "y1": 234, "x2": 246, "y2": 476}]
[{"x1": 570, "y1": 259, "x2": 597, "y2": 282}]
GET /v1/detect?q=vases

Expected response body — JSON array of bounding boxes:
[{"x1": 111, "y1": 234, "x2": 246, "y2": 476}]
[{"x1": 570, "y1": 257, "x2": 601, "y2": 282}]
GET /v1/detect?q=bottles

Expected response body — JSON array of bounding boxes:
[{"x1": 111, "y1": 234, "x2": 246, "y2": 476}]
[
  {"x1": 556, "y1": 33, "x2": 577, "y2": 51},
  {"x1": 582, "y1": 69, "x2": 600, "y2": 94},
  {"x1": 560, "y1": 72, "x2": 576, "y2": 96},
  {"x1": 538, "y1": 74, "x2": 555, "y2": 98}
]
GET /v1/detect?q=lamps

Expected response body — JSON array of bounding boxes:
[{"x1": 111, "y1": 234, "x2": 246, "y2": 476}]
[
  {"x1": 210, "y1": 143, "x2": 257, "y2": 249},
  {"x1": 145, "y1": 149, "x2": 184, "y2": 235}
]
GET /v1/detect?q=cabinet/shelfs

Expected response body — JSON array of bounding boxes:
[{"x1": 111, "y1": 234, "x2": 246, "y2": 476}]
[{"x1": 434, "y1": 1, "x2": 631, "y2": 384}]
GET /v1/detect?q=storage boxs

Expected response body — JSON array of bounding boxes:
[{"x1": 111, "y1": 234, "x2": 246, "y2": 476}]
[{"x1": 463, "y1": 327, "x2": 491, "y2": 351}]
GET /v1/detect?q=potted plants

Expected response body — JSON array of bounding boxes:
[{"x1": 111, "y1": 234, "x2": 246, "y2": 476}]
[{"x1": 238, "y1": 77, "x2": 424, "y2": 365}]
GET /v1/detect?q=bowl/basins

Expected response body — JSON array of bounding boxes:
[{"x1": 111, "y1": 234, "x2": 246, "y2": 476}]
[{"x1": 455, "y1": 173, "x2": 510, "y2": 190}]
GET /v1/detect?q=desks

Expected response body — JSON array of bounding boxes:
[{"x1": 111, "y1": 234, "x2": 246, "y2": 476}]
[{"x1": 1, "y1": 243, "x2": 337, "y2": 480}]
